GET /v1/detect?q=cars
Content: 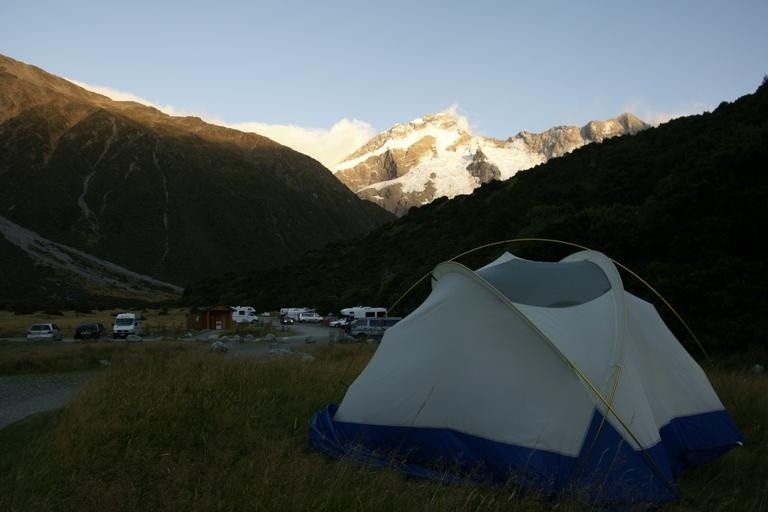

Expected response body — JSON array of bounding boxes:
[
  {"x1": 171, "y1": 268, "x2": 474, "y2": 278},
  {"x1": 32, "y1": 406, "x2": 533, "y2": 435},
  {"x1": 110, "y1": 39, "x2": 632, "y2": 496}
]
[
  {"x1": 27, "y1": 322, "x2": 63, "y2": 342},
  {"x1": 75, "y1": 322, "x2": 106, "y2": 340}
]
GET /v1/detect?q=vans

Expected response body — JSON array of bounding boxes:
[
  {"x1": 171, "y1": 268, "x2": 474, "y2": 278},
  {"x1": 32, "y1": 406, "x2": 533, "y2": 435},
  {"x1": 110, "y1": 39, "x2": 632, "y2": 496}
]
[
  {"x1": 261, "y1": 304, "x2": 405, "y2": 343},
  {"x1": 231, "y1": 305, "x2": 259, "y2": 326},
  {"x1": 111, "y1": 313, "x2": 139, "y2": 338}
]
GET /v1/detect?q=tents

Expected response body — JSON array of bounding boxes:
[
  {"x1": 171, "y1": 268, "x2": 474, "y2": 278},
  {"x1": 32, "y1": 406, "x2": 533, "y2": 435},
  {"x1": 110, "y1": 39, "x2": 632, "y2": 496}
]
[{"x1": 309, "y1": 248, "x2": 743, "y2": 507}]
